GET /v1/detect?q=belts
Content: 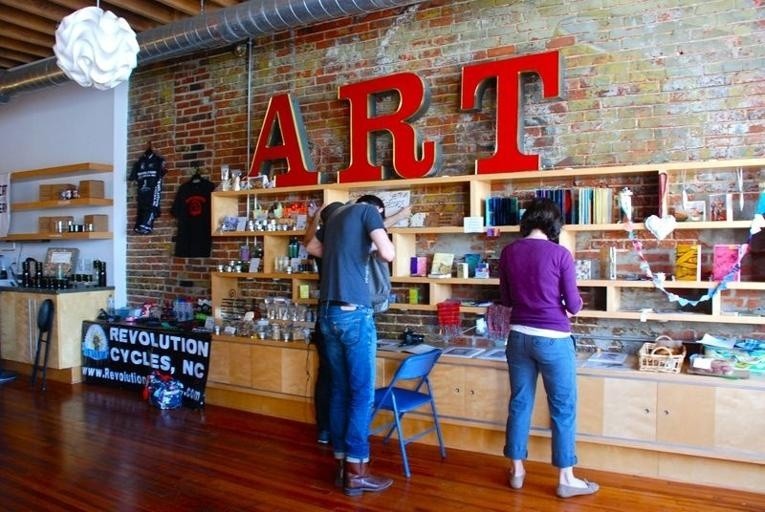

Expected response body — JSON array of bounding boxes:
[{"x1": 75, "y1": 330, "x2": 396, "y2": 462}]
[{"x1": 318, "y1": 299, "x2": 363, "y2": 307}]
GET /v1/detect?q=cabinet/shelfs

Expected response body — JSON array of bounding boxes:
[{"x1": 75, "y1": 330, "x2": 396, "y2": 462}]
[
  {"x1": 0, "y1": 287, "x2": 116, "y2": 384},
  {"x1": 0, "y1": 162, "x2": 113, "y2": 241},
  {"x1": 204, "y1": 312, "x2": 765, "y2": 493},
  {"x1": 202, "y1": 154, "x2": 765, "y2": 335}
]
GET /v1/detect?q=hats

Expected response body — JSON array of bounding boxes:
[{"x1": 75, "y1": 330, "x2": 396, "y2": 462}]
[{"x1": 319, "y1": 200, "x2": 351, "y2": 228}]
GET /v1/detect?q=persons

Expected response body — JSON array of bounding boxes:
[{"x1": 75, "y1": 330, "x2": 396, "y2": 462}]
[
  {"x1": 305, "y1": 193, "x2": 396, "y2": 497},
  {"x1": 314, "y1": 201, "x2": 418, "y2": 445},
  {"x1": 500, "y1": 200, "x2": 600, "y2": 497}
]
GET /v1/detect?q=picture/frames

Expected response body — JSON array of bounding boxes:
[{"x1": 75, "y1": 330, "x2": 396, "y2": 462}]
[{"x1": 43, "y1": 246, "x2": 79, "y2": 275}]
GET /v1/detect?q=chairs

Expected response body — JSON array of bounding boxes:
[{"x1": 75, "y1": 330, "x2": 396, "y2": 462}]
[{"x1": 366, "y1": 349, "x2": 447, "y2": 476}]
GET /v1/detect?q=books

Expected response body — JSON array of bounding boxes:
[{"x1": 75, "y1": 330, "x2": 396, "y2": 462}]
[
  {"x1": 482, "y1": 185, "x2": 616, "y2": 228},
  {"x1": 473, "y1": 346, "x2": 507, "y2": 363},
  {"x1": 376, "y1": 338, "x2": 402, "y2": 351},
  {"x1": 427, "y1": 253, "x2": 454, "y2": 279},
  {"x1": 588, "y1": 346, "x2": 627, "y2": 371},
  {"x1": 712, "y1": 244, "x2": 742, "y2": 282},
  {"x1": 402, "y1": 341, "x2": 444, "y2": 356},
  {"x1": 674, "y1": 243, "x2": 702, "y2": 282},
  {"x1": 437, "y1": 345, "x2": 486, "y2": 359}
]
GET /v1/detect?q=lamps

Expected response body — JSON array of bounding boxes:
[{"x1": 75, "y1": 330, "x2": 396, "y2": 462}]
[{"x1": 54, "y1": 0, "x2": 142, "y2": 92}]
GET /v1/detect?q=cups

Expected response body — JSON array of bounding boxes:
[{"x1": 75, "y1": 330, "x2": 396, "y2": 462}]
[{"x1": 429, "y1": 319, "x2": 464, "y2": 343}]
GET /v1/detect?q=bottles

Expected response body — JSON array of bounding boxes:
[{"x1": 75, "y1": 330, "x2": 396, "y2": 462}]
[
  {"x1": 210, "y1": 298, "x2": 324, "y2": 346},
  {"x1": 212, "y1": 234, "x2": 263, "y2": 275},
  {"x1": 273, "y1": 237, "x2": 319, "y2": 275},
  {"x1": 246, "y1": 195, "x2": 299, "y2": 232},
  {"x1": 55, "y1": 189, "x2": 95, "y2": 234},
  {"x1": 107, "y1": 295, "x2": 114, "y2": 321},
  {"x1": 618, "y1": 187, "x2": 633, "y2": 223},
  {"x1": 171, "y1": 291, "x2": 196, "y2": 324},
  {"x1": 23, "y1": 259, "x2": 109, "y2": 290}
]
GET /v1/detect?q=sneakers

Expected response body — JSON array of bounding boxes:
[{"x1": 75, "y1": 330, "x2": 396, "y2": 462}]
[
  {"x1": 506, "y1": 466, "x2": 526, "y2": 489},
  {"x1": 557, "y1": 478, "x2": 600, "y2": 498},
  {"x1": 318, "y1": 429, "x2": 329, "y2": 444}
]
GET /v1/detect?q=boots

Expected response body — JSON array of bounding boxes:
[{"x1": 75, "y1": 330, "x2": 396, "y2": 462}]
[
  {"x1": 343, "y1": 462, "x2": 393, "y2": 496},
  {"x1": 333, "y1": 460, "x2": 345, "y2": 487}
]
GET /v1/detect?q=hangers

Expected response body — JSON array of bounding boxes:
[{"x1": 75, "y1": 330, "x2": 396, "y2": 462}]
[
  {"x1": 190, "y1": 162, "x2": 201, "y2": 182},
  {"x1": 144, "y1": 140, "x2": 152, "y2": 157}
]
